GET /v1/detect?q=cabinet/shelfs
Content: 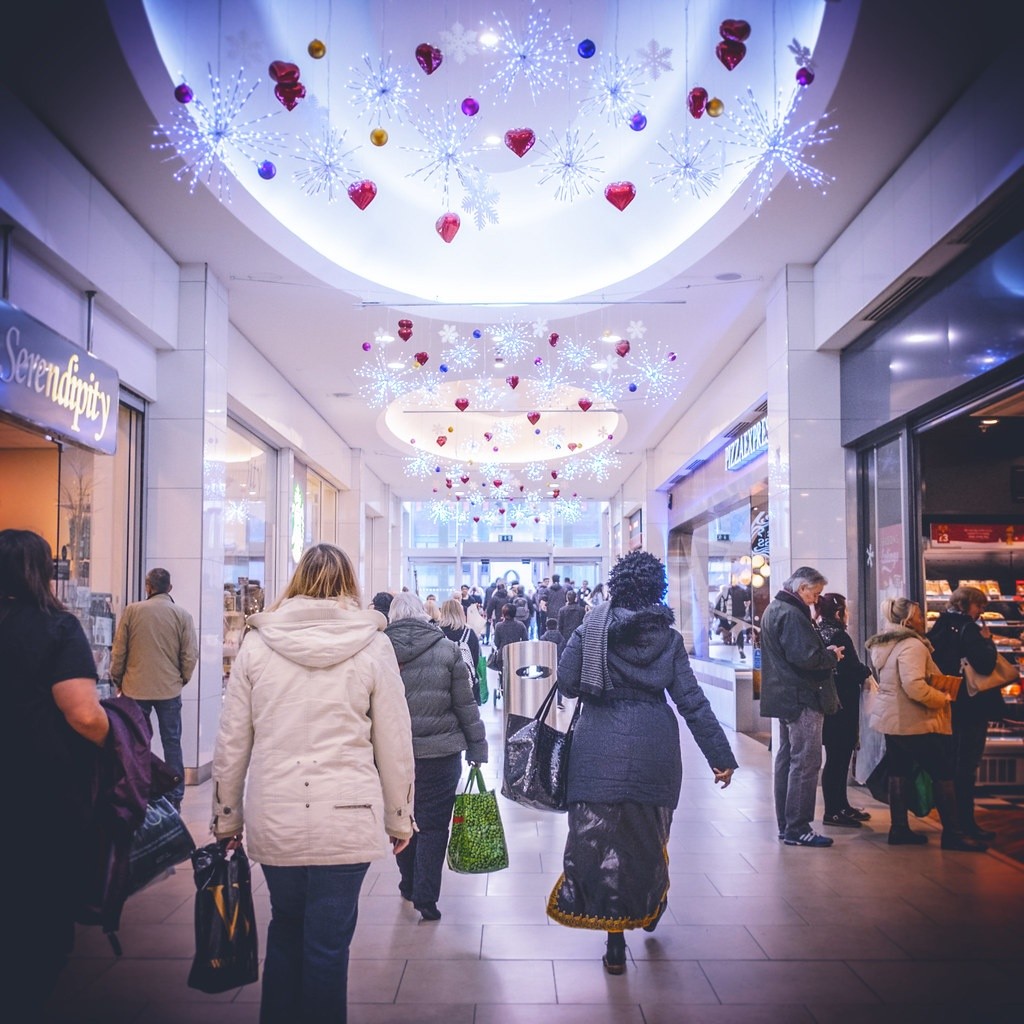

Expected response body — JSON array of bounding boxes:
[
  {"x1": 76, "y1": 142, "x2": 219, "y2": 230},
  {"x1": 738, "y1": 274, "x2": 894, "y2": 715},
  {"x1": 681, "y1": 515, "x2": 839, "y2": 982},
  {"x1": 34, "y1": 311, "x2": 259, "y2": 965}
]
[{"x1": 920, "y1": 546, "x2": 1024, "y2": 661}]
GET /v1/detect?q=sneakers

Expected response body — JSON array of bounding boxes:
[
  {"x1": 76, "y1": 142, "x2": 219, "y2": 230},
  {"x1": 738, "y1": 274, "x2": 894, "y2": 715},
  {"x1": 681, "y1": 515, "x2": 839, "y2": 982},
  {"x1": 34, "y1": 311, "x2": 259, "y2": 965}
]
[
  {"x1": 822, "y1": 809, "x2": 861, "y2": 827},
  {"x1": 843, "y1": 807, "x2": 870, "y2": 821},
  {"x1": 784, "y1": 830, "x2": 833, "y2": 848},
  {"x1": 778, "y1": 830, "x2": 785, "y2": 839}
]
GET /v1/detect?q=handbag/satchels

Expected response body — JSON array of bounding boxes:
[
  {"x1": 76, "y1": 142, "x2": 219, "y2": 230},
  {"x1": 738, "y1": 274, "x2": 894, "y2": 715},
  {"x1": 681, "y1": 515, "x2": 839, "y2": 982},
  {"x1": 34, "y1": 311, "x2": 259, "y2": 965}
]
[
  {"x1": 187, "y1": 838, "x2": 261, "y2": 993},
  {"x1": 476, "y1": 649, "x2": 489, "y2": 704},
  {"x1": 122, "y1": 795, "x2": 194, "y2": 896},
  {"x1": 501, "y1": 681, "x2": 582, "y2": 813},
  {"x1": 865, "y1": 752, "x2": 936, "y2": 817},
  {"x1": 959, "y1": 621, "x2": 1018, "y2": 697},
  {"x1": 447, "y1": 766, "x2": 508, "y2": 874},
  {"x1": 488, "y1": 647, "x2": 503, "y2": 671},
  {"x1": 816, "y1": 678, "x2": 842, "y2": 716}
]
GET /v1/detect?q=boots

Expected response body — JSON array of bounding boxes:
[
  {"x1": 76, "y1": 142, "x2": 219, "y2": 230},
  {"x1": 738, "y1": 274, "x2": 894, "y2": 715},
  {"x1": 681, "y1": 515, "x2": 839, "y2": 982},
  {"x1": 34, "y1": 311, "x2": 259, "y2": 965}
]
[
  {"x1": 957, "y1": 773, "x2": 997, "y2": 839},
  {"x1": 602, "y1": 933, "x2": 627, "y2": 975},
  {"x1": 887, "y1": 776, "x2": 928, "y2": 844},
  {"x1": 933, "y1": 781, "x2": 987, "y2": 852},
  {"x1": 644, "y1": 899, "x2": 668, "y2": 931}
]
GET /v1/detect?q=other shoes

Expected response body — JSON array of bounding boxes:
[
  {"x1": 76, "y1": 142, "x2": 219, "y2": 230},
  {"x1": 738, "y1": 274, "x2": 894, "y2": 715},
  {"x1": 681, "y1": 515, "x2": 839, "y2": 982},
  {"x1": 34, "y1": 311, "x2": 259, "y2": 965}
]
[
  {"x1": 400, "y1": 891, "x2": 414, "y2": 900},
  {"x1": 414, "y1": 901, "x2": 441, "y2": 920}
]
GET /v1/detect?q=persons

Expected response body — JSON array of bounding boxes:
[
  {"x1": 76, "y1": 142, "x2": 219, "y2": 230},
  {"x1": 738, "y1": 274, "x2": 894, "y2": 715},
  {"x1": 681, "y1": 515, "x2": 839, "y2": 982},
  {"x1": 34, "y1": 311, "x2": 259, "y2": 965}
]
[
  {"x1": 421, "y1": 574, "x2": 608, "y2": 645},
  {"x1": 714, "y1": 572, "x2": 769, "y2": 648},
  {"x1": 865, "y1": 598, "x2": 988, "y2": 851},
  {"x1": 368, "y1": 592, "x2": 394, "y2": 624},
  {"x1": 438, "y1": 599, "x2": 482, "y2": 709},
  {"x1": 814, "y1": 592, "x2": 873, "y2": 828},
  {"x1": 760, "y1": 567, "x2": 846, "y2": 848},
  {"x1": 557, "y1": 551, "x2": 739, "y2": 976},
  {"x1": 539, "y1": 619, "x2": 568, "y2": 710},
  {"x1": 110, "y1": 568, "x2": 200, "y2": 813},
  {"x1": 926, "y1": 587, "x2": 1009, "y2": 840},
  {"x1": 1, "y1": 529, "x2": 115, "y2": 1023},
  {"x1": 386, "y1": 591, "x2": 488, "y2": 921},
  {"x1": 210, "y1": 543, "x2": 420, "y2": 1024},
  {"x1": 495, "y1": 603, "x2": 530, "y2": 695}
]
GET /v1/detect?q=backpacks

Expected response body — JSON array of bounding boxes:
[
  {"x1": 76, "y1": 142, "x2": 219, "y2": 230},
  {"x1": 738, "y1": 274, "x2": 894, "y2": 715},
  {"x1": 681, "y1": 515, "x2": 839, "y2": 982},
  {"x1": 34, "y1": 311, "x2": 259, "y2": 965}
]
[
  {"x1": 438, "y1": 624, "x2": 476, "y2": 690},
  {"x1": 512, "y1": 597, "x2": 529, "y2": 621}
]
